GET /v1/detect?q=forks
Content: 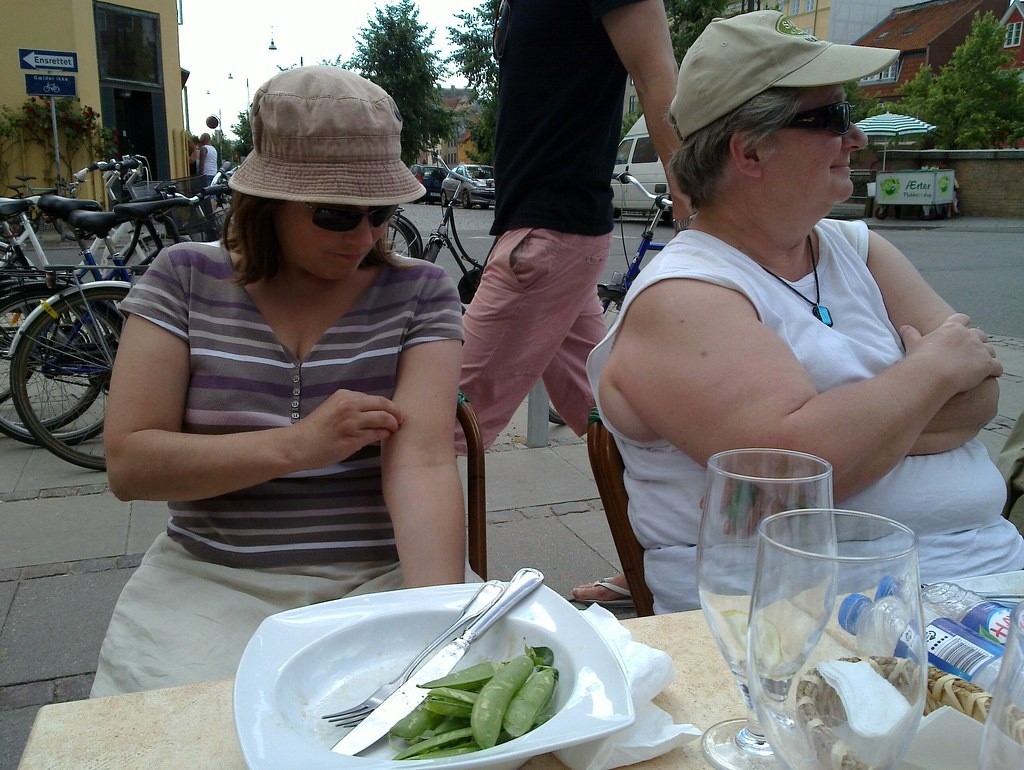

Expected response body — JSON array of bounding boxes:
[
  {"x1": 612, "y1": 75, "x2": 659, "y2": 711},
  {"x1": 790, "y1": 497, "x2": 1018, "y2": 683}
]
[{"x1": 321, "y1": 580, "x2": 504, "y2": 730}]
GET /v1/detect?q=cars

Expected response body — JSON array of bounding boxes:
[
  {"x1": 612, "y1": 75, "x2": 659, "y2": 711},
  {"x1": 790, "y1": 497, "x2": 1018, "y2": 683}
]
[
  {"x1": 409, "y1": 165, "x2": 449, "y2": 206},
  {"x1": 442, "y1": 163, "x2": 496, "y2": 210}
]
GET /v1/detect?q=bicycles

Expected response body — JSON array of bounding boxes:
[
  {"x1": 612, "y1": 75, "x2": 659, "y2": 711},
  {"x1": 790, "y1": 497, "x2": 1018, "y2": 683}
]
[{"x1": 0, "y1": 149, "x2": 673, "y2": 469}]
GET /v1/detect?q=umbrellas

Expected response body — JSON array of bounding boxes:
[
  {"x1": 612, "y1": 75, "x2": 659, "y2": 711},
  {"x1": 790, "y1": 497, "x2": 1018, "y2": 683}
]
[{"x1": 853, "y1": 111, "x2": 938, "y2": 172}]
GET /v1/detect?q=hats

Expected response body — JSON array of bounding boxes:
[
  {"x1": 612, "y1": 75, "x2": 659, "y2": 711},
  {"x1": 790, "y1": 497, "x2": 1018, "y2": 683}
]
[
  {"x1": 668, "y1": 12, "x2": 901, "y2": 144},
  {"x1": 228, "y1": 67, "x2": 427, "y2": 206}
]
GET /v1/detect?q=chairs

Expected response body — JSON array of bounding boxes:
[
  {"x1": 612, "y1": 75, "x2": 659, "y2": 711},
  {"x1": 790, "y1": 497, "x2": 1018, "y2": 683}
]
[{"x1": 451, "y1": 396, "x2": 656, "y2": 616}]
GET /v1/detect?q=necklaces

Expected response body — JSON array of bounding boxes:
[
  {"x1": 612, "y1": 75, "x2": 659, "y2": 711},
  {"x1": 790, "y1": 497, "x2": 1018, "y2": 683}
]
[{"x1": 760, "y1": 233, "x2": 834, "y2": 327}]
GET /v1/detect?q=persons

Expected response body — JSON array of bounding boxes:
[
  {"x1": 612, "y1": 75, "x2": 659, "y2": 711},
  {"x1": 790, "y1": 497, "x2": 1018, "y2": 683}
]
[
  {"x1": 87, "y1": 64, "x2": 465, "y2": 696},
  {"x1": 584, "y1": 8, "x2": 1023, "y2": 613},
  {"x1": 453, "y1": 1, "x2": 696, "y2": 610},
  {"x1": 187, "y1": 132, "x2": 218, "y2": 213}
]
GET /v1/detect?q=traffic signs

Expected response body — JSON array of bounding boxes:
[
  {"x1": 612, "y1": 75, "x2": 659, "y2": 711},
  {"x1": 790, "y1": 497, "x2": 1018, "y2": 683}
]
[{"x1": 19, "y1": 48, "x2": 77, "y2": 73}]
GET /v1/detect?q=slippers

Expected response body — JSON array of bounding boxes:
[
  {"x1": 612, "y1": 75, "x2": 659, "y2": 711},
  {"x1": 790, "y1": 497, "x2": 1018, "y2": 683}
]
[{"x1": 574, "y1": 575, "x2": 634, "y2": 608}]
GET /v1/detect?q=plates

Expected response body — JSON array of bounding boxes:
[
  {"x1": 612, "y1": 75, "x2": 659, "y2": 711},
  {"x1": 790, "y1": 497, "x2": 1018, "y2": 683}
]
[{"x1": 233, "y1": 580, "x2": 636, "y2": 769}]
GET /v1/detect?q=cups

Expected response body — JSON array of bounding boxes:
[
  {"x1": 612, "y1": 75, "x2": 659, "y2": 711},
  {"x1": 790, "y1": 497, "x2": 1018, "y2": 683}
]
[
  {"x1": 746, "y1": 508, "x2": 928, "y2": 770},
  {"x1": 980, "y1": 601, "x2": 1023, "y2": 770}
]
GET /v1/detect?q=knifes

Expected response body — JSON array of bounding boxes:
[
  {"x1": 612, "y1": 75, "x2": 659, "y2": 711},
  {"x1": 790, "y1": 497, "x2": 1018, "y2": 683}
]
[{"x1": 330, "y1": 567, "x2": 545, "y2": 756}]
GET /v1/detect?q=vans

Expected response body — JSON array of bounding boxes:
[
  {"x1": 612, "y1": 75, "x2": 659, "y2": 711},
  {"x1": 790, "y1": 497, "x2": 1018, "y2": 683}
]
[{"x1": 611, "y1": 113, "x2": 673, "y2": 226}]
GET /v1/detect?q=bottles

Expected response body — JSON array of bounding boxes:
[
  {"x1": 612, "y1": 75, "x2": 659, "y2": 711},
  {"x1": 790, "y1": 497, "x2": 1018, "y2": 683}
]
[
  {"x1": 871, "y1": 573, "x2": 1024, "y2": 656},
  {"x1": 838, "y1": 595, "x2": 1024, "y2": 705}
]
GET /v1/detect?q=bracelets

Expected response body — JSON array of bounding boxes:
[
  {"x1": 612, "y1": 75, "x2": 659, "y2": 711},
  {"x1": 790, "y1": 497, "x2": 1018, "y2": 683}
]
[{"x1": 672, "y1": 213, "x2": 695, "y2": 232}]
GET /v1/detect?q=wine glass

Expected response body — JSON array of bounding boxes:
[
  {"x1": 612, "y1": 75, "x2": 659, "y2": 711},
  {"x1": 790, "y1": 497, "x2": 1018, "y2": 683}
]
[{"x1": 695, "y1": 448, "x2": 839, "y2": 770}]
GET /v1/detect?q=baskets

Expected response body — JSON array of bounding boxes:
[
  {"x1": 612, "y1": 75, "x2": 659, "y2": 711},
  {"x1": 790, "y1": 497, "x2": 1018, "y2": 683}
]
[
  {"x1": 184, "y1": 206, "x2": 230, "y2": 241},
  {"x1": 130, "y1": 179, "x2": 162, "y2": 199}
]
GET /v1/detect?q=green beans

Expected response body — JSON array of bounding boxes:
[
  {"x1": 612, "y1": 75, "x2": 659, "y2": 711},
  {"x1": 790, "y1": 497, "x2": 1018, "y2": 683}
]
[{"x1": 389, "y1": 653, "x2": 557, "y2": 761}]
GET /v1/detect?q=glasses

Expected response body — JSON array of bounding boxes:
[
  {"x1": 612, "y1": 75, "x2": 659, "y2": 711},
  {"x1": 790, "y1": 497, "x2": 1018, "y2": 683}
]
[
  {"x1": 754, "y1": 100, "x2": 851, "y2": 136},
  {"x1": 305, "y1": 196, "x2": 401, "y2": 230}
]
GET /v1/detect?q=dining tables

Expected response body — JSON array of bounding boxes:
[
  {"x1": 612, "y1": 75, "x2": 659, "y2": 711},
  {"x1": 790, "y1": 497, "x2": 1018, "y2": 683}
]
[{"x1": 16, "y1": 587, "x2": 887, "y2": 770}]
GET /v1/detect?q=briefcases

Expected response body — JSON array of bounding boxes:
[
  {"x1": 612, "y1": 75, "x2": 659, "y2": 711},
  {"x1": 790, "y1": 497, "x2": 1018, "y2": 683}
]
[{"x1": 155, "y1": 174, "x2": 213, "y2": 232}]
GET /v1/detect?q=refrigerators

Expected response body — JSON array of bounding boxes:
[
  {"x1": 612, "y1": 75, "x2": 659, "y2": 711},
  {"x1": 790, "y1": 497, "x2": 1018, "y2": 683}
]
[{"x1": 875, "y1": 169, "x2": 955, "y2": 205}]
